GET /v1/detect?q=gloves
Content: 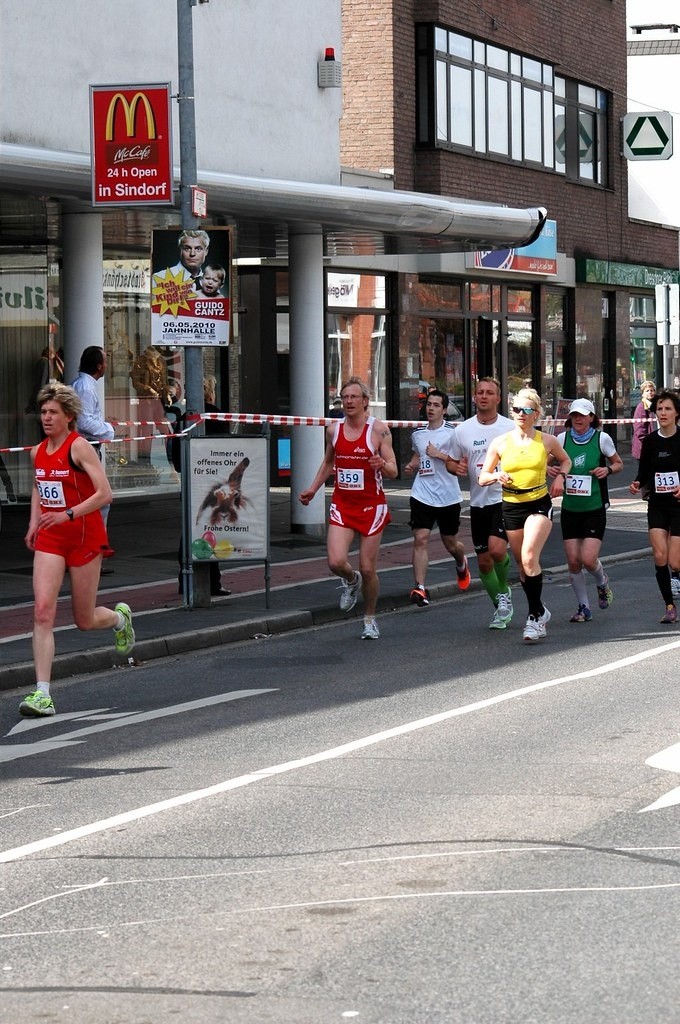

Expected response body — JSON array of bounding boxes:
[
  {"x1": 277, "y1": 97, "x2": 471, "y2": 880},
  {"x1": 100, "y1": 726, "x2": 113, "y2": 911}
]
[{"x1": 24, "y1": 397, "x2": 36, "y2": 415}]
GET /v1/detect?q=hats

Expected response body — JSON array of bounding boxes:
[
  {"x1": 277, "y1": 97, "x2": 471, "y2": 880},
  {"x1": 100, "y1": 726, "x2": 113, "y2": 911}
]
[{"x1": 568, "y1": 397, "x2": 596, "y2": 416}]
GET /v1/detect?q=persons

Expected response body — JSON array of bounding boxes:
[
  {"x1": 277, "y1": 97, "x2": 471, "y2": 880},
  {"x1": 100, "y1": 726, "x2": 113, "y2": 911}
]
[
  {"x1": 18, "y1": 384, "x2": 134, "y2": 718},
  {"x1": 151, "y1": 230, "x2": 210, "y2": 301},
  {"x1": 478, "y1": 389, "x2": 572, "y2": 639},
  {"x1": 35, "y1": 349, "x2": 49, "y2": 385},
  {"x1": 171, "y1": 375, "x2": 231, "y2": 595},
  {"x1": 299, "y1": 378, "x2": 398, "y2": 641},
  {"x1": 191, "y1": 265, "x2": 226, "y2": 301},
  {"x1": 132, "y1": 347, "x2": 173, "y2": 468},
  {"x1": 54, "y1": 346, "x2": 63, "y2": 383},
  {"x1": 629, "y1": 389, "x2": 680, "y2": 624},
  {"x1": 631, "y1": 381, "x2": 657, "y2": 459},
  {"x1": 403, "y1": 389, "x2": 470, "y2": 606},
  {"x1": 546, "y1": 399, "x2": 623, "y2": 623},
  {"x1": 72, "y1": 346, "x2": 116, "y2": 532},
  {"x1": 446, "y1": 377, "x2": 520, "y2": 629},
  {"x1": 666, "y1": 565, "x2": 680, "y2": 597}
]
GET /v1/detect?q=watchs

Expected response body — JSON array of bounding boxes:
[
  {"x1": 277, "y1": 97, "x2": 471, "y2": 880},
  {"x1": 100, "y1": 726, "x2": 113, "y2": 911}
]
[
  {"x1": 557, "y1": 471, "x2": 568, "y2": 480},
  {"x1": 606, "y1": 466, "x2": 612, "y2": 475},
  {"x1": 66, "y1": 508, "x2": 74, "y2": 521}
]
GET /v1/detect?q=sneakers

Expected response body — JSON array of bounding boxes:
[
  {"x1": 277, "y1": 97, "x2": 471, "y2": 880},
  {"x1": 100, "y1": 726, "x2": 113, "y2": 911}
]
[
  {"x1": 112, "y1": 602, "x2": 135, "y2": 656},
  {"x1": 596, "y1": 573, "x2": 614, "y2": 609},
  {"x1": 537, "y1": 603, "x2": 552, "y2": 638},
  {"x1": 570, "y1": 603, "x2": 593, "y2": 622},
  {"x1": 410, "y1": 585, "x2": 430, "y2": 607},
  {"x1": 522, "y1": 614, "x2": 540, "y2": 641},
  {"x1": 488, "y1": 608, "x2": 512, "y2": 629},
  {"x1": 671, "y1": 577, "x2": 680, "y2": 598},
  {"x1": 19, "y1": 690, "x2": 56, "y2": 715},
  {"x1": 660, "y1": 604, "x2": 679, "y2": 623},
  {"x1": 497, "y1": 586, "x2": 514, "y2": 620},
  {"x1": 361, "y1": 617, "x2": 379, "y2": 640},
  {"x1": 340, "y1": 570, "x2": 363, "y2": 612},
  {"x1": 455, "y1": 554, "x2": 471, "y2": 590}
]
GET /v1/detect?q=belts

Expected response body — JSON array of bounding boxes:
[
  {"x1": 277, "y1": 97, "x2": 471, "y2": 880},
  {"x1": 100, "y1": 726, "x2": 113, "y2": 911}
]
[{"x1": 87, "y1": 439, "x2": 102, "y2": 450}]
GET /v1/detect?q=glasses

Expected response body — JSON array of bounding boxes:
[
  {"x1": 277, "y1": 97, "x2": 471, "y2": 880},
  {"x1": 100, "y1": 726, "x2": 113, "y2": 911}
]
[{"x1": 512, "y1": 406, "x2": 540, "y2": 415}]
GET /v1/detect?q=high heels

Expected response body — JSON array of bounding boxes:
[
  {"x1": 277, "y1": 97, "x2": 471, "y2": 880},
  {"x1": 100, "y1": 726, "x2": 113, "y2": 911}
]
[{"x1": 6, "y1": 488, "x2": 18, "y2": 505}]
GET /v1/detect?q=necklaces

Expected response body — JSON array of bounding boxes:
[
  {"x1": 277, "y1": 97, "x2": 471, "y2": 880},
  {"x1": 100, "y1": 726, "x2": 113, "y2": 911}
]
[{"x1": 476, "y1": 414, "x2": 498, "y2": 423}]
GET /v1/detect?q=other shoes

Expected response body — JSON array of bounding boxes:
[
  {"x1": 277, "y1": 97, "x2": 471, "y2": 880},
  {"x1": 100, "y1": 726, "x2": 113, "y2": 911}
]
[
  {"x1": 100, "y1": 567, "x2": 114, "y2": 574},
  {"x1": 211, "y1": 586, "x2": 232, "y2": 595}
]
